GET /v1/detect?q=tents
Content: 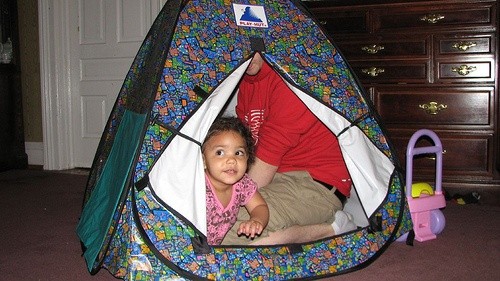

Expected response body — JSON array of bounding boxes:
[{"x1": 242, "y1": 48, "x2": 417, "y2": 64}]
[{"x1": 74, "y1": 1, "x2": 416, "y2": 281}]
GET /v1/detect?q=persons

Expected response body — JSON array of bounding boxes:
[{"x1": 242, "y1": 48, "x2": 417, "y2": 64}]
[
  {"x1": 219, "y1": 51, "x2": 357, "y2": 246},
  {"x1": 201, "y1": 118, "x2": 270, "y2": 247}
]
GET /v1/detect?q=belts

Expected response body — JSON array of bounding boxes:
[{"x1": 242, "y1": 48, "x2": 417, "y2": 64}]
[{"x1": 312, "y1": 179, "x2": 346, "y2": 205}]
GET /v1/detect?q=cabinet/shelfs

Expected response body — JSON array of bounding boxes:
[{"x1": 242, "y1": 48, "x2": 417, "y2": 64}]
[{"x1": 299, "y1": 0, "x2": 499, "y2": 201}]
